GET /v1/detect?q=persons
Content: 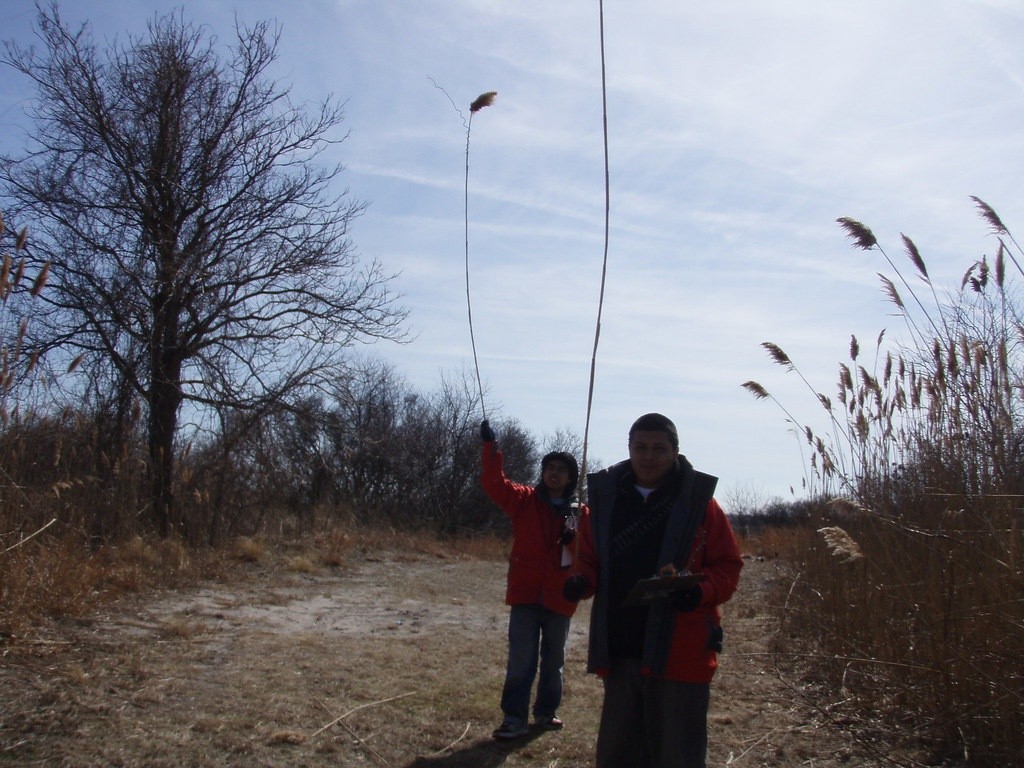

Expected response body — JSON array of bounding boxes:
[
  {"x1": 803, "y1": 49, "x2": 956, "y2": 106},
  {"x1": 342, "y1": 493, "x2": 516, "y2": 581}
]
[
  {"x1": 561, "y1": 413, "x2": 744, "y2": 768},
  {"x1": 480, "y1": 420, "x2": 589, "y2": 740}
]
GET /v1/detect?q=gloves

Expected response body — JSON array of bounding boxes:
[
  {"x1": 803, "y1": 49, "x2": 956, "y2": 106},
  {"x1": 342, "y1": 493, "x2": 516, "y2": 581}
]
[
  {"x1": 563, "y1": 577, "x2": 586, "y2": 601},
  {"x1": 559, "y1": 521, "x2": 574, "y2": 544},
  {"x1": 480, "y1": 421, "x2": 496, "y2": 442},
  {"x1": 653, "y1": 586, "x2": 700, "y2": 616}
]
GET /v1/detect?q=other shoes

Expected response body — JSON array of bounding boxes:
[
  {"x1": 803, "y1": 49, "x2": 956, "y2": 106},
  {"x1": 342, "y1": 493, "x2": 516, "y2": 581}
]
[
  {"x1": 492, "y1": 723, "x2": 529, "y2": 738},
  {"x1": 535, "y1": 714, "x2": 563, "y2": 729}
]
